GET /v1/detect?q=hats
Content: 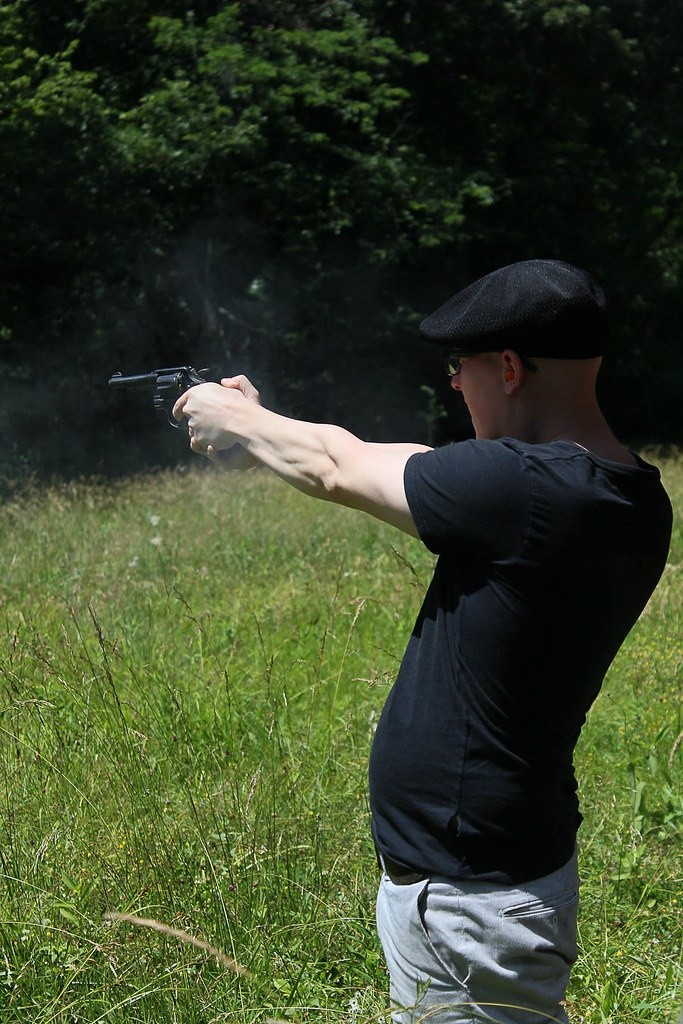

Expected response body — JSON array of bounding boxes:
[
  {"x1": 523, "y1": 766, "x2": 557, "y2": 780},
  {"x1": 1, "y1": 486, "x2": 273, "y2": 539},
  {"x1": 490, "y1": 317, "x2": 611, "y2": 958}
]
[{"x1": 419, "y1": 260, "x2": 610, "y2": 359}]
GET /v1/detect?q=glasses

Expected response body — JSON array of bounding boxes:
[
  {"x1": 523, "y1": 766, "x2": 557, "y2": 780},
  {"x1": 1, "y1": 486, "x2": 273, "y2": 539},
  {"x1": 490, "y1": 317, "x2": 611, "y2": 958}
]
[{"x1": 445, "y1": 349, "x2": 537, "y2": 376}]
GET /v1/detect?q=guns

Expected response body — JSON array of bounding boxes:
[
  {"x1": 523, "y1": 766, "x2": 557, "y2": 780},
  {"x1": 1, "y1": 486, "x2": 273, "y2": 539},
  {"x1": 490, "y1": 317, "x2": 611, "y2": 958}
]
[{"x1": 108, "y1": 366, "x2": 205, "y2": 432}]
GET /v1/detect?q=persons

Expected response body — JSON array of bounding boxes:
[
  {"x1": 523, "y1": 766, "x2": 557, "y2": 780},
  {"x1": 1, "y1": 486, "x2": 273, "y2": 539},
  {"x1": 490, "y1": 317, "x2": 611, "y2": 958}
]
[{"x1": 170, "y1": 257, "x2": 673, "y2": 1024}]
